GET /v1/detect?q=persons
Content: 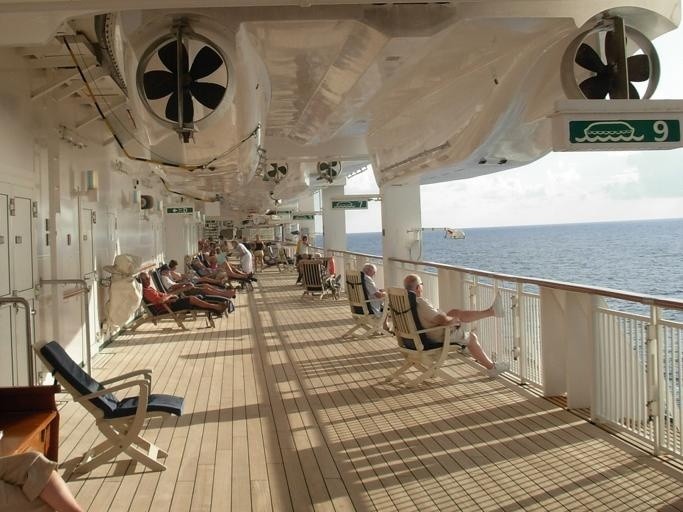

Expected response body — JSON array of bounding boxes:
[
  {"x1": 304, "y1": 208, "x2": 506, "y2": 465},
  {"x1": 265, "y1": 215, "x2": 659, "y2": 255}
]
[
  {"x1": 402, "y1": 273, "x2": 510, "y2": 379},
  {"x1": 168, "y1": 259, "x2": 227, "y2": 286},
  {"x1": 137, "y1": 271, "x2": 228, "y2": 314},
  {"x1": 158, "y1": 263, "x2": 237, "y2": 300},
  {"x1": 0, "y1": 452, "x2": 83, "y2": 512},
  {"x1": 359, "y1": 262, "x2": 390, "y2": 337},
  {"x1": 183, "y1": 232, "x2": 341, "y2": 300}
]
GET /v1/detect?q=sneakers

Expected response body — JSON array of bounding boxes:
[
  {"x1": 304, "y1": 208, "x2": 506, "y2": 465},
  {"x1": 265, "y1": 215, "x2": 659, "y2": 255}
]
[
  {"x1": 381, "y1": 328, "x2": 395, "y2": 335},
  {"x1": 491, "y1": 290, "x2": 507, "y2": 317},
  {"x1": 488, "y1": 360, "x2": 510, "y2": 380}
]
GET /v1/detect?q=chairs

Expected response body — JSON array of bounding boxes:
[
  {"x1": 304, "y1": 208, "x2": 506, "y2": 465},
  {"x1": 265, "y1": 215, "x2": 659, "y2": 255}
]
[
  {"x1": 275, "y1": 248, "x2": 465, "y2": 388},
  {"x1": 29, "y1": 339, "x2": 185, "y2": 478},
  {"x1": 128, "y1": 250, "x2": 256, "y2": 331}
]
